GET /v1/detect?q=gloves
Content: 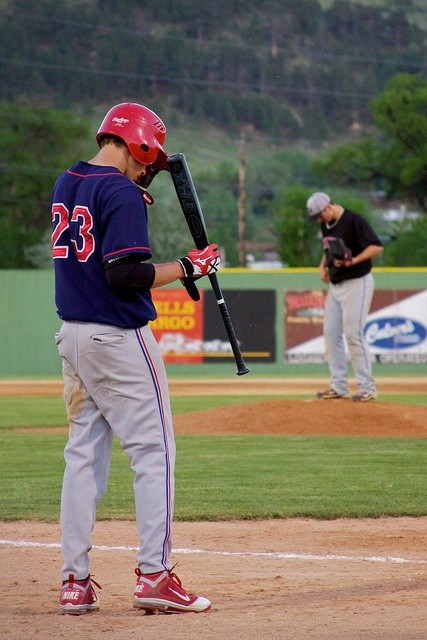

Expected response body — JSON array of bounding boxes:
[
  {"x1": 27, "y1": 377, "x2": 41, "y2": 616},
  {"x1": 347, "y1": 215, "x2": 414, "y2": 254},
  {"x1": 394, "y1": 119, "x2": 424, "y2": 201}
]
[
  {"x1": 322, "y1": 238, "x2": 351, "y2": 269},
  {"x1": 177, "y1": 243, "x2": 221, "y2": 306}
]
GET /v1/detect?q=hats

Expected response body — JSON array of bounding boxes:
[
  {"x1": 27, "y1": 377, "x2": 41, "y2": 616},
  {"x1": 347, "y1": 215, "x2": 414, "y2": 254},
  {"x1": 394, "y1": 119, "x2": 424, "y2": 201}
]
[{"x1": 307, "y1": 192, "x2": 331, "y2": 222}]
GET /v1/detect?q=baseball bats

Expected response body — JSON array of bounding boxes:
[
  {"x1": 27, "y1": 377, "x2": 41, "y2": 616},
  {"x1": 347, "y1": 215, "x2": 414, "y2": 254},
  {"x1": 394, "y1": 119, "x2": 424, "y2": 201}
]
[{"x1": 165, "y1": 153, "x2": 250, "y2": 376}]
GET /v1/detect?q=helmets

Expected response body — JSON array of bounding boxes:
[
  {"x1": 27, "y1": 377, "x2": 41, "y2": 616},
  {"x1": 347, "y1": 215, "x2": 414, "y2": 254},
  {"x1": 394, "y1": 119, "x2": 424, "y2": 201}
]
[{"x1": 98, "y1": 102, "x2": 171, "y2": 163}]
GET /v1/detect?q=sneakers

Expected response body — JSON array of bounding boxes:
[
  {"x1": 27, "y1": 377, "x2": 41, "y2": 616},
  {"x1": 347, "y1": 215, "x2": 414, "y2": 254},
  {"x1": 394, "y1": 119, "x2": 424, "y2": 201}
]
[
  {"x1": 353, "y1": 391, "x2": 376, "y2": 401},
  {"x1": 317, "y1": 389, "x2": 349, "y2": 399},
  {"x1": 59, "y1": 574, "x2": 102, "y2": 614},
  {"x1": 133, "y1": 562, "x2": 211, "y2": 613}
]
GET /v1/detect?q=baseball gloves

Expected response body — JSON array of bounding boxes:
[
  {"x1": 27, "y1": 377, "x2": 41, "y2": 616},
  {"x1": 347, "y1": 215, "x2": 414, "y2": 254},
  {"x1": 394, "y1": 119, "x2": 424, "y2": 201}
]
[{"x1": 322, "y1": 235, "x2": 352, "y2": 285}]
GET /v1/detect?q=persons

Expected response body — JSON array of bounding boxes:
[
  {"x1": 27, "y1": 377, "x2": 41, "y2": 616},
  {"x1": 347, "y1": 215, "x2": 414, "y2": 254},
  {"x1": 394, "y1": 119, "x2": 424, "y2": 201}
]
[
  {"x1": 51, "y1": 103, "x2": 221, "y2": 614},
  {"x1": 307, "y1": 191, "x2": 384, "y2": 403}
]
